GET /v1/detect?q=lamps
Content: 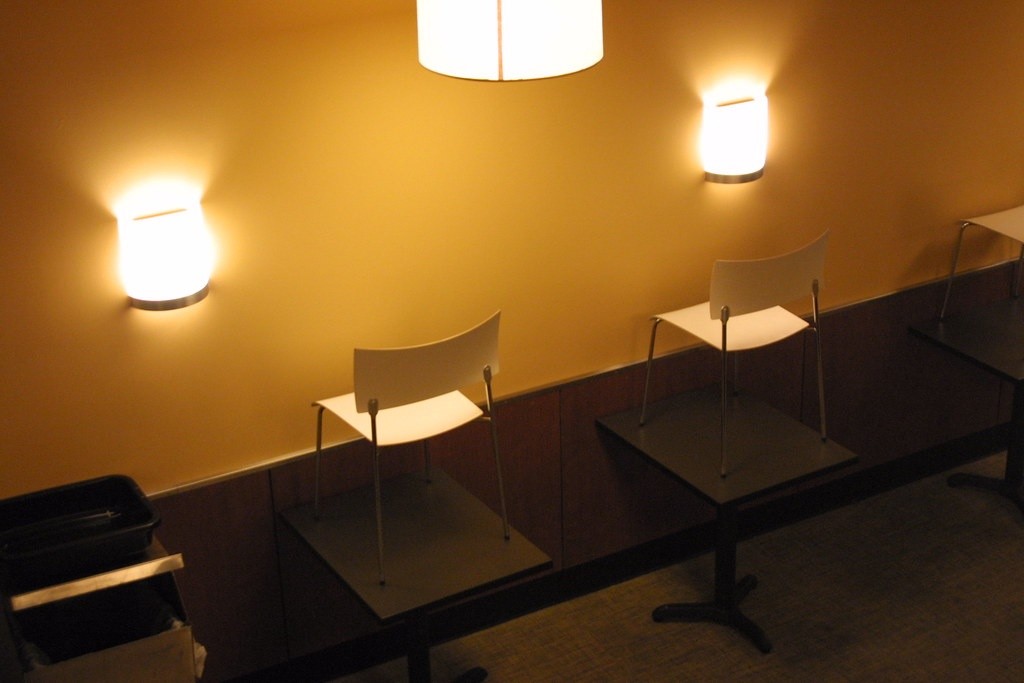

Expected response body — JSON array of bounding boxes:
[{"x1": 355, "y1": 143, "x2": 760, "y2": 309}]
[
  {"x1": 417, "y1": 0, "x2": 603, "y2": 81},
  {"x1": 699, "y1": 94, "x2": 769, "y2": 184},
  {"x1": 117, "y1": 181, "x2": 215, "y2": 311}
]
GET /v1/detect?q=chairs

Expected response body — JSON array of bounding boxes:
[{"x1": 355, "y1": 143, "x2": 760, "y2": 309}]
[
  {"x1": 311, "y1": 310, "x2": 510, "y2": 586},
  {"x1": 936, "y1": 205, "x2": 1024, "y2": 321},
  {"x1": 638, "y1": 228, "x2": 829, "y2": 479}
]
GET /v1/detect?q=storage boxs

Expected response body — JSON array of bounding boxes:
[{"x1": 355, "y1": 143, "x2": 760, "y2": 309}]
[{"x1": 0, "y1": 474, "x2": 188, "y2": 665}]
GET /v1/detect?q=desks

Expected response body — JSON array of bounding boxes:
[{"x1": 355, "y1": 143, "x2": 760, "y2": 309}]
[
  {"x1": 595, "y1": 380, "x2": 860, "y2": 653},
  {"x1": 280, "y1": 469, "x2": 553, "y2": 683},
  {"x1": 909, "y1": 297, "x2": 1024, "y2": 517}
]
